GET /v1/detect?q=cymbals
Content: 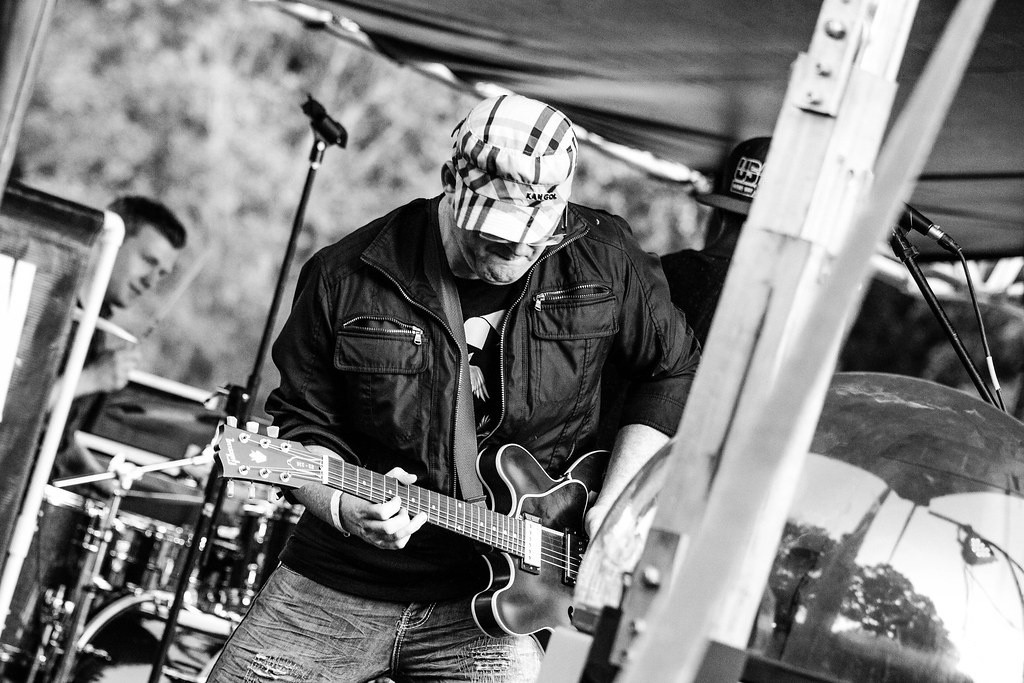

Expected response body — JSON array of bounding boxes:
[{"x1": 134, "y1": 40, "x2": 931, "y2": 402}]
[{"x1": 71, "y1": 303, "x2": 146, "y2": 346}]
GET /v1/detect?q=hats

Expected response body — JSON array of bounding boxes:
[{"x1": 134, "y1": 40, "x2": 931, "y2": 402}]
[
  {"x1": 697, "y1": 138, "x2": 772, "y2": 216},
  {"x1": 451, "y1": 94, "x2": 579, "y2": 244}
]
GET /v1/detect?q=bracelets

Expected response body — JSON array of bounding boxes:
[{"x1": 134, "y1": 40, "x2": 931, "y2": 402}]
[{"x1": 329, "y1": 489, "x2": 350, "y2": 538}]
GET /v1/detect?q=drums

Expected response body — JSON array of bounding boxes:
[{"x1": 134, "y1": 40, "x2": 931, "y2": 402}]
[
  {"x1": 62, "y1": 587, "x2": 231, "y2": 683},
  {"x1": 206, "y1": 479, "x2": 307, "y2": 613},
  {"x1": 98, "y1": 504, "x2": 236, "y2": 601}
]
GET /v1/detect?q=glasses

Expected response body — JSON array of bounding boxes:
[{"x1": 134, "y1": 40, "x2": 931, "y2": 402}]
[{"x1": 479, "y1": 202, "x2": 569, "y2": 246}]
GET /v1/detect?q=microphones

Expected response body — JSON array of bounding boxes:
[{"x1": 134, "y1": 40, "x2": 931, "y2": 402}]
[{"x1": 896, "y1": 202, "x2": 963, "y2": 252}]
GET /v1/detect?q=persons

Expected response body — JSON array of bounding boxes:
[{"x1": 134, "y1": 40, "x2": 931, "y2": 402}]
[
  {"x1": 27, "y1": 195, "x2": 186, "y2": 483},
  {"x1": 665, "y1": 137, "x2": 771, "y2": 346},
  {"x1": 203, "y1": 92, "x2": 702, "y2": 682}
]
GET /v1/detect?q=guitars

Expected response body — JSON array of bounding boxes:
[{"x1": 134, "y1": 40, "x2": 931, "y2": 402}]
[{"x1": 213, "y1": 413, "x2": 610, "y2": 639}]
